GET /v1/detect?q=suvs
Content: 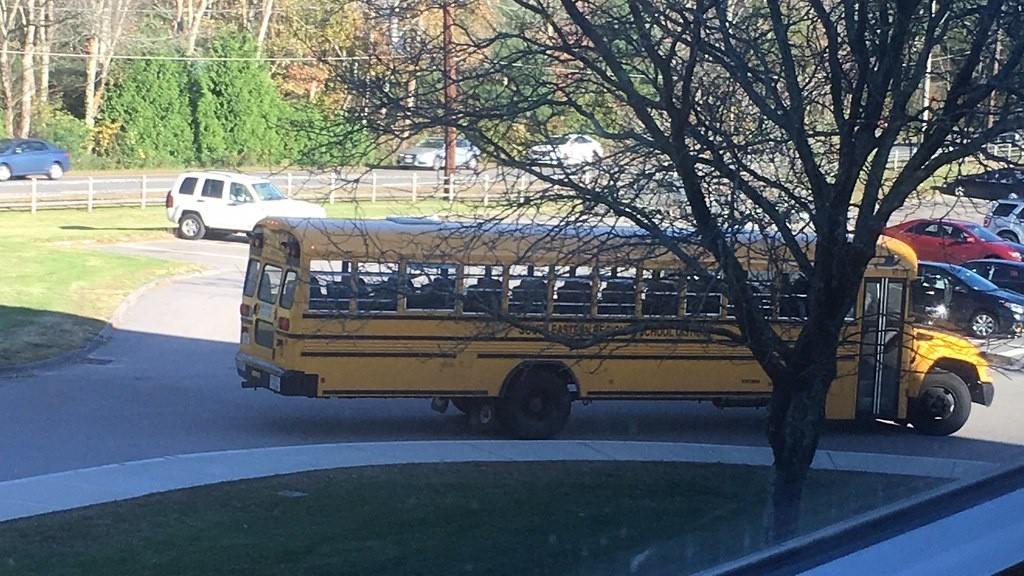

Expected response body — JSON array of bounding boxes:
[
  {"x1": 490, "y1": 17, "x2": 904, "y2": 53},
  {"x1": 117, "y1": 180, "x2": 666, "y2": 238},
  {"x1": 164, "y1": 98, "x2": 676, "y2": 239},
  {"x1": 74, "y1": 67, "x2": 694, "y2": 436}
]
[
  {"x1": 983, "y1": 197, "x2": 1024, "y2": 244},
  {"x1": 165, "y1": 169, "x2": 326, "y2": 242}
]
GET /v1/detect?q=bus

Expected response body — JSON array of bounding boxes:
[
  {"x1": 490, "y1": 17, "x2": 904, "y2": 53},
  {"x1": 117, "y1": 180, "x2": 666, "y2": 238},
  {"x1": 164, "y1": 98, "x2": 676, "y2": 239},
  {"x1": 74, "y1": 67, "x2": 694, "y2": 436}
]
[{"x1": 231, "y1": 216, "x2": 995, "y2": 440}]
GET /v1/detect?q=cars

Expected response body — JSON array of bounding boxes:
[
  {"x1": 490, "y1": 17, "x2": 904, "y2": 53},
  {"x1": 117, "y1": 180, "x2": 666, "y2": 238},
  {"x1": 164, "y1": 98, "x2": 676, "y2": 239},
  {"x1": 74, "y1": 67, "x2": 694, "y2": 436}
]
[
  {"x1": 0, "y1": 137, "x2": 72, "y2": 181},
  {"x1": 941, "y1": 168, "x2": 1024, "y2": 200},
  {"x1": 866, "y1": 261, "x2": 1023, "y2": 338},
  {"x1": 526, "y1": 132, "x2": 603, "y2": 166},
  {"x1": 882, "y1": 219, "x2": 1024, "y2": 262},
  {"x1": 960, "y1": 258, "x2": 1023, "y2": 294},
  {"x1": 395, "y1": 136, "x2": 483, "y2": 170}
]
